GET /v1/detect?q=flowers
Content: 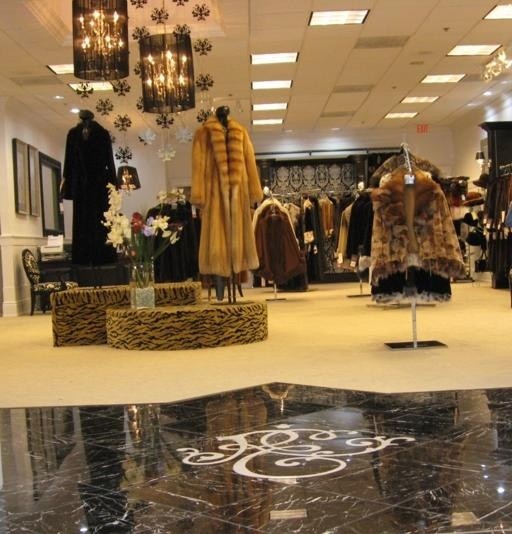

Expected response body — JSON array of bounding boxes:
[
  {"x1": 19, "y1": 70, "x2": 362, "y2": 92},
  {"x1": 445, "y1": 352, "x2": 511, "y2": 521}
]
[{"x1": 101, "y1": 183, "x2": 187, "y2": 288}]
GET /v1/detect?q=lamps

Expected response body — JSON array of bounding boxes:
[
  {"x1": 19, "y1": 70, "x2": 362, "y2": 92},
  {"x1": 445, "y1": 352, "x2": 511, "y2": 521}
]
[
  {"x1": 117, "y1": 167, "x2": 141, "y2": 192},
  {"x1": 72, "y1": 0, "x2": 128, "y2": 82},
  {"x1": 138, "y1": 0, "x2": 195, "y2": 114}
]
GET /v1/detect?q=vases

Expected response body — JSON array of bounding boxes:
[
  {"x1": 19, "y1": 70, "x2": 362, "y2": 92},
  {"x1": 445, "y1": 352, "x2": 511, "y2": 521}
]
[{"x1": 129, "y1": 256, "x2": 155, "y2": 310}]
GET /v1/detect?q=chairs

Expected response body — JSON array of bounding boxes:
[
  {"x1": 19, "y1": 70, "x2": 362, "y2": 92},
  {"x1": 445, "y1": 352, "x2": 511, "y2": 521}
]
[{"x1": 22, "y1": 249, "x2": 78, "y2": 316}]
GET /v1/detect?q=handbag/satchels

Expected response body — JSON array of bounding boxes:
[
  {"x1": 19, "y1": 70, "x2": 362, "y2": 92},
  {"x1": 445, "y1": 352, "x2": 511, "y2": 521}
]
[
  {"x1": 475, "y1": 259, "x2": 491, "y2": 272},
  {"x1": 467, "y1": 232, "x2": 484, "y2": 246}
]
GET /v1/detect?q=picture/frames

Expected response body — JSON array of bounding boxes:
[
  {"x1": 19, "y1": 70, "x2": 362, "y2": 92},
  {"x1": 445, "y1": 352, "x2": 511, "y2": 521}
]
[{"x1": 12, "y1": 138, "x2": 41, "y2": 217}]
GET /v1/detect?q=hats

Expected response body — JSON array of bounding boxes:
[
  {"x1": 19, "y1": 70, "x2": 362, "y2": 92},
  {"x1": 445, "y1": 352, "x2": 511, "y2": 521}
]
[
  {"x1": 473, "y1": 173, "x2": 489, "y2": 188},
  {"x1": 462, "y1": 191, "x2": 482, "y2": 206},
  {"x1": 460, "y1": 213, "x2": 478, "y2": 228}
]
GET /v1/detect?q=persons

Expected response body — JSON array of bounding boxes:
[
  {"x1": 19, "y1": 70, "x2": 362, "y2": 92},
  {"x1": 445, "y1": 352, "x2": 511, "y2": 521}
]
[
  {"x1": 203, "y1": 386, "x2": 277, "y2": 534},
  {"x1": 62, "y1": 108, "x2": 124, "y2": 267},
  {"x1": 74, "y1": 404, "x2": 130, "y2": 496},
  {"x1": 192, "y1": 104, "x2": 261, "y2": 295}
]
